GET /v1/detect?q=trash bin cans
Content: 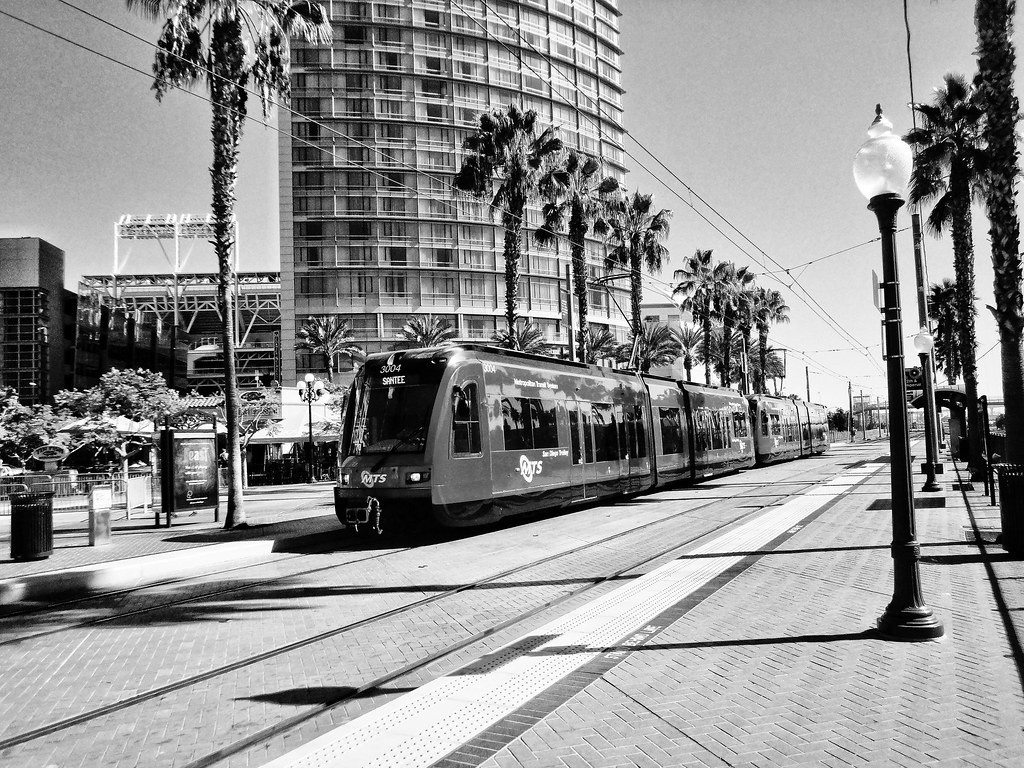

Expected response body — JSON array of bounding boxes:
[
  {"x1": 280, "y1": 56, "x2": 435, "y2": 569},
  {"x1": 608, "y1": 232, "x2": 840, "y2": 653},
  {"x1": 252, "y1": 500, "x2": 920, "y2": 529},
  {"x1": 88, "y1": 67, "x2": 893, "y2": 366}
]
[
  {"x1": 991, "y1": 462, "x2": 1024, "y2": 557},
  {"x1": 8, "y1": 491, "x2": 56, "y2": 558}
]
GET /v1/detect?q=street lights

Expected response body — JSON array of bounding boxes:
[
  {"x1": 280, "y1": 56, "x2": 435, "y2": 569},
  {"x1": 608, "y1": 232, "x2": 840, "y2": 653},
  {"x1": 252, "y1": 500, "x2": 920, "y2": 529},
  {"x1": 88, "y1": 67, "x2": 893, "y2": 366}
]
[
  {"x1": 852, "y1": 105, "x2": 945, "y2": 643},
  {"x1": 297, "y1": 373, "x2": 324, "y2": 484},
  {"x1": 914, "y1": 325, "x2": 944, "y2": 492}
]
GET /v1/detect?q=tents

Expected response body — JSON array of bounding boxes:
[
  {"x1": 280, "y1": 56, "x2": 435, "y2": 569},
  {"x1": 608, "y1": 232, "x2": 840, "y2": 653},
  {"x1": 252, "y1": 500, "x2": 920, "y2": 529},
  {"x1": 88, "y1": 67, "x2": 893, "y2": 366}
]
[{"x1": 240, "y1": 408, "x2": 342, "y2": 489}]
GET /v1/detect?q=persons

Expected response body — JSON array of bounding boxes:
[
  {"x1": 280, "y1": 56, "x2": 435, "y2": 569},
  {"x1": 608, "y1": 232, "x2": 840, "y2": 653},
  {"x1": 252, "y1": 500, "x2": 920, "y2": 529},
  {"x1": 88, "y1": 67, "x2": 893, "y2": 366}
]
[
  {"x1": 220, "y1": 449, "x2": 229, "y2": 465},
  {"x1": 881, "y1": 423, "x2": 887, "y2": 429},
  {"x1": 866, "y1": 419, "x2": 877, "y2": 430}
]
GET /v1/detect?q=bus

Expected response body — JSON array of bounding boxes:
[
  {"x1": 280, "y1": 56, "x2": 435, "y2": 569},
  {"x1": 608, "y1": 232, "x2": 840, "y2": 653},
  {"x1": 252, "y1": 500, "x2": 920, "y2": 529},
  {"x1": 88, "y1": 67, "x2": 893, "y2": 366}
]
[{"x1": 334, "y1": 344, "x2": 831, "y2": 544}]
